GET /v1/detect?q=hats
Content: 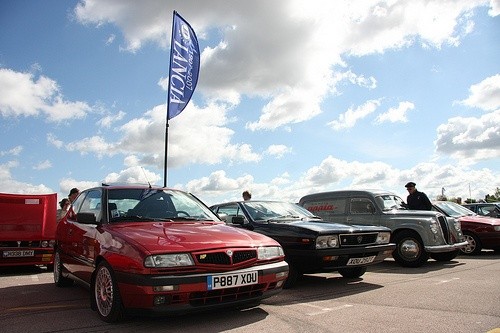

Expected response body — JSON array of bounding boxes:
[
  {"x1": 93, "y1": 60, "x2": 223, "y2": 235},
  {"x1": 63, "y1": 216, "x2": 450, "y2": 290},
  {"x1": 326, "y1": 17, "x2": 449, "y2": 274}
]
[
  {"x1": 68, "y1": 188, "x2": 79, "y2": 196},
  {"x1": 405, "y1": 182, "x2": 416, "y2": 186},
  {"x1": 59, "y1": 199, "x2": 71, "y2": 205}
]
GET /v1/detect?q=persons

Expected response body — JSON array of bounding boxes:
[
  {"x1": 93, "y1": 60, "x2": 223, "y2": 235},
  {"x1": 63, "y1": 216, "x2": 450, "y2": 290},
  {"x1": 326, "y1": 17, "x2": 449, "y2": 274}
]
[
  {"x1": 56, "y1": 198, "x2": 70, "y2": 224},
  {"x1": 68, "y1": 188, "x2": 80, "y2": 203},
  {"x1": 400, "y1": 182, "x2": 433, "y2": 211}
]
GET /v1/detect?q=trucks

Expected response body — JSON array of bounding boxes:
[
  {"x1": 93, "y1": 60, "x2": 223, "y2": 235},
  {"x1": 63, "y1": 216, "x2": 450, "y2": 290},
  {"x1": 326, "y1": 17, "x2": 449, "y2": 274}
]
[{"x1": 0, "y1": 193, "x2": 57, "y2": 272}]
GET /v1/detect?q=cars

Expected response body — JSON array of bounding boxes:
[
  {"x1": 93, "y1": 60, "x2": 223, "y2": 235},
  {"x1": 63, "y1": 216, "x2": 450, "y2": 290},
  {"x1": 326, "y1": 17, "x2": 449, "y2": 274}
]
[
  {"x1": 298, "y1": 189, "x2": 470, "y2": 268},
  {"x1": 430, "y1": 201, "x2": 500, "y2": 256},
  {"x1": 209, "y1": 201, "x2": 396, "y2": 278},
  {"x1": 53, "y1": 167, "x2": 289, "y2": 322},
  {"x1": 461, "y1": 203, "x2": 500, "y2": 219}
]
